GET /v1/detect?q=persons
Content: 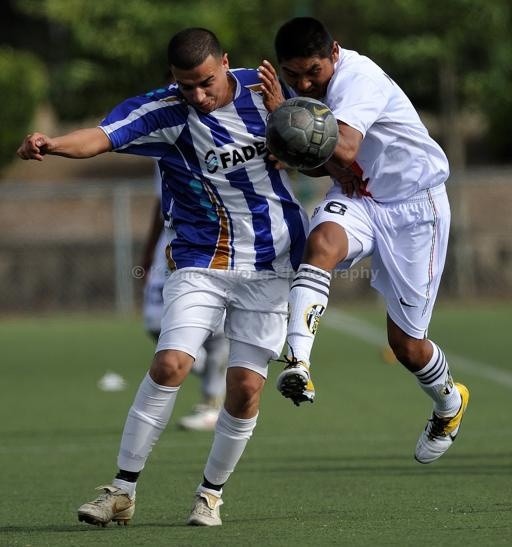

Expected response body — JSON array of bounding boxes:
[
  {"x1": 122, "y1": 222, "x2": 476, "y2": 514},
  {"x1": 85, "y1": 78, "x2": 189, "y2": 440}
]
[
  {"x1": 135, "y1": 159, "x2": 231, "y2": 432},
  {"x1": 14, "y1": 26, "x2": 310, "y2": 529},
  {"x1": 256, "y1": 15, "x2": 473, "y2": 465}
]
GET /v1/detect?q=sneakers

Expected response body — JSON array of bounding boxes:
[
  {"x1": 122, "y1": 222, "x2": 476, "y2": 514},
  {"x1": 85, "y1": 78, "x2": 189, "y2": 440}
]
[
  {"x1": 178, "y1": 403, "x2": 221, "y2": 431},
  {"x1": 186, "y1": 491, "x2": 224, "y2": 527},
  {"x1": 412, "y1": 381, "x2": 469, "y2": 464},
  {"x1": 277, "y1": 359, "x2": 315, "y2": 406},
  {"x1": 77, "y1": 483, "x2": 137, "y2": 527}
]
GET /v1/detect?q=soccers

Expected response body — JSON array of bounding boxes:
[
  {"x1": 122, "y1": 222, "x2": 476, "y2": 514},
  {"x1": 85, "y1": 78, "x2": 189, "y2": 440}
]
[{"x1": 265, "y1": 97, "x2": 339, "y2": 169}]
